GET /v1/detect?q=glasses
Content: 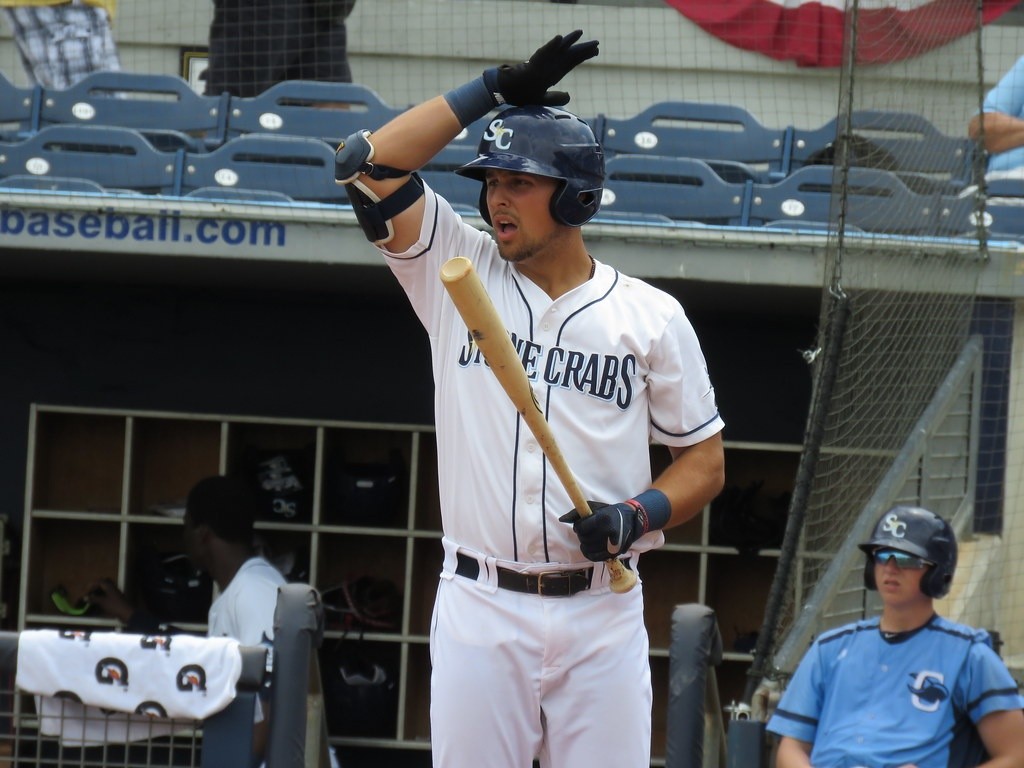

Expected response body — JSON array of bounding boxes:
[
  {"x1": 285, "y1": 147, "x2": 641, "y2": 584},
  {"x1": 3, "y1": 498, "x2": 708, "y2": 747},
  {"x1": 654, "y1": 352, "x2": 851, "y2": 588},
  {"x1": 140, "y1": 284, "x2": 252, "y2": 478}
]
[{"x1": 872, "y1": 551, "x2": 936, "y2": 570}]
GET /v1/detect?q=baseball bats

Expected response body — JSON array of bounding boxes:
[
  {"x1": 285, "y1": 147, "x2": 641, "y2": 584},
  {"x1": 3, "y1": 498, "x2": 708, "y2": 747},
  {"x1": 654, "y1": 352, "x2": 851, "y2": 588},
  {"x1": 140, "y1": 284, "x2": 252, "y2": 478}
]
[{"x1": 438, "y1": 256, "x2": 640, "y2": 594}]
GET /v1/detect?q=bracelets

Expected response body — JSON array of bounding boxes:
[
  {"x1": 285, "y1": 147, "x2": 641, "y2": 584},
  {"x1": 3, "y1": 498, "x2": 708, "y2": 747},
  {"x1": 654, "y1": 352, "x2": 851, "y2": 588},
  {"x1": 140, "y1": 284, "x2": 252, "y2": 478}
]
[
  {"x1": 633, "y1": 489, "x2": 671, "y2": 532},
  {"x1": 444, "y1": 77, "x2": 495, "y2": 129}
]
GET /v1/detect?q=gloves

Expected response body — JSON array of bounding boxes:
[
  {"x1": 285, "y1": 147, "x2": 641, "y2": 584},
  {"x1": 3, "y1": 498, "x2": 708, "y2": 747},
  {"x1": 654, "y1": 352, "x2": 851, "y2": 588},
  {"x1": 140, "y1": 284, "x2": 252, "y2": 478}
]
[
  {"x1": 484, "y1": 31, "x2": 599, "y2": 106},
  {"x1": 560, "y1": 501, "x2": 644, "y2": 562}
]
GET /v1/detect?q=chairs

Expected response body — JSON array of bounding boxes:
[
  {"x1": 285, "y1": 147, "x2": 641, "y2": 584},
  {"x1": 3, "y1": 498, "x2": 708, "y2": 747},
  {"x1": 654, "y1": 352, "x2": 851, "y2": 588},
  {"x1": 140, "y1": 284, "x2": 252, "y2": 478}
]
[{"x1": 0, "y1": 71, "x2": 1024, "y2": 244}]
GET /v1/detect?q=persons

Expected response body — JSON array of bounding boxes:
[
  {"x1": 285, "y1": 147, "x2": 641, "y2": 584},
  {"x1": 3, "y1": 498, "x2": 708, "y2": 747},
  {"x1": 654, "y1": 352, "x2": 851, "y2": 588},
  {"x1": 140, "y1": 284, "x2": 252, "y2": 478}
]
[
  {"x1": 83, "y1": 476, "x2": 338, "y2": 768},
  {"x1": 0, "y1": 1, "x2": 123, "y2": 96},
  {"x1": 331, "y1": 29, "x2": 724, "y2": 768},
  {"x1": 207, "y1": 0, "x2": 356, "y2": 109},
  {"x1": 968, "y1": 53, "x2": 1023, "y2": 170},
  {"x1": 765, "y1": 507, "x2": 1023, "y2": 768}
]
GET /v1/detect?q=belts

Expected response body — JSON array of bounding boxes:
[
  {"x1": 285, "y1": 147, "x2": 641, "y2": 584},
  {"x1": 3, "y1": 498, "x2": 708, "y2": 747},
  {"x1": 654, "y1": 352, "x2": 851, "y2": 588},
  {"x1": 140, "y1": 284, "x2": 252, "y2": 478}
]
[{"x1": 456, "y1": 552, "x2": 631, "y2": 596}]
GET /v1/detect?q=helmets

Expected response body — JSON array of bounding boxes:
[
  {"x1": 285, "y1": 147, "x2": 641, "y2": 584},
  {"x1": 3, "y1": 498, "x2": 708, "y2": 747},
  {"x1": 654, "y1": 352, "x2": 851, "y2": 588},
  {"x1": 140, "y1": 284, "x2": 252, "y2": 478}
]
[
  {"x1": 455, "y1": 105, "x2": 604, "y2": 226},
  {"x1": 860, "y1": 505, "x2": 954, "y2": 596}
]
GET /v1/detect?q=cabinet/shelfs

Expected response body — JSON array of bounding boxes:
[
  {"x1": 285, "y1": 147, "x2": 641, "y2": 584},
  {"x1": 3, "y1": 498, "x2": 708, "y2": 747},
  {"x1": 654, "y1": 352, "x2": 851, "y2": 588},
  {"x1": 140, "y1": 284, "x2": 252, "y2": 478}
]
[{"x1": 18, "y1": 404, "x2": 899, "y2": 767}]
